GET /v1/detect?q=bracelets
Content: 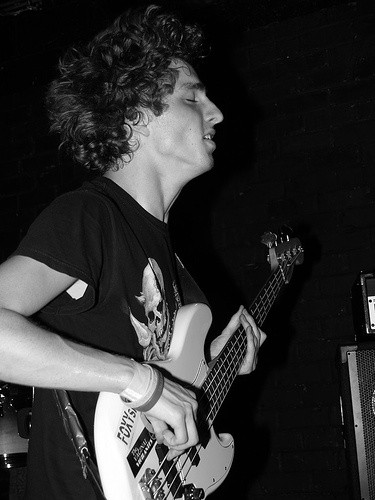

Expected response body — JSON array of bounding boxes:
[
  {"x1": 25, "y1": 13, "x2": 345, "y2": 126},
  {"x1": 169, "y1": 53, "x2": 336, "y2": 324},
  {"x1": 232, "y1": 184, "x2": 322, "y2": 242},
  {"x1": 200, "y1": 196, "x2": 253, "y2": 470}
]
[
  {"x1": 130, "y1": 369, "x2": 165, "y2": 412},
  {"x1": 121, "y1": 364, "x2": 159, "y2": 408}
]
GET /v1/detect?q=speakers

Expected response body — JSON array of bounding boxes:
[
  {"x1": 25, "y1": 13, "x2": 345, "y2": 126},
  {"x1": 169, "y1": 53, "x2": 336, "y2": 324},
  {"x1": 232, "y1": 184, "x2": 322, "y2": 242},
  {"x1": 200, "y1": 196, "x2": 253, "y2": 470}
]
[{"x1": 335, "y1": 340, "x2": 375, "y2": 499}]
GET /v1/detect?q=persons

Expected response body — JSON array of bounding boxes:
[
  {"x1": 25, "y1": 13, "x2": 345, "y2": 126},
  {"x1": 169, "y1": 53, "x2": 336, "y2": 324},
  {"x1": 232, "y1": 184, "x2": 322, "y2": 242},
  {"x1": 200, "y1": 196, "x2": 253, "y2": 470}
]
[{"x1": 0, "y1": 0, "x2": 268, "y2": 499}]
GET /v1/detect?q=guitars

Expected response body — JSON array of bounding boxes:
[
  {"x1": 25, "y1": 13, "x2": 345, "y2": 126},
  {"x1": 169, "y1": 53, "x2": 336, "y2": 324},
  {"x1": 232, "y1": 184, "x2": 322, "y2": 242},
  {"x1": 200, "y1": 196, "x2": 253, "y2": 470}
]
[{"x1": 94, "y1": 227, "x2": 305, "y2": 500}]
